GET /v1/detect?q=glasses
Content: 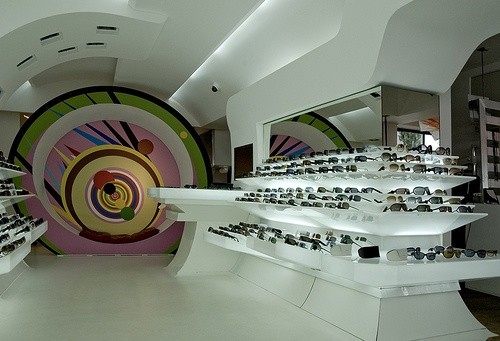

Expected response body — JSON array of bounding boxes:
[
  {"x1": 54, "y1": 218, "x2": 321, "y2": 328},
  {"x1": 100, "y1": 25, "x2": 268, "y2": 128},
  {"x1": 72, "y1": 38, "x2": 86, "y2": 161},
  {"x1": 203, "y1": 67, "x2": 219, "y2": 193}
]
[
  {"x1": 428, "y1": 245, "x2": 453, "y2": 254},
  {"x1": 406, "y1": 247, "x2": 420, "y2": 256},
  {"x1": 389, "y1": 164, "x2": 405, "y2": 171},
  {"x1": 414, "y1": 251, "x2": 439, "y2": 260},
  {"x1": 443, "y1": 250, "x2": 461, "y2": 259},
  {"x1": 410, "y1": 186, "x2": 431, "y2": 195},
  {"x1": 380, "y1": 153, "x2": 401, "y2": 162},
  {"x1": 461, "y1": 248, "x2": 486, "y2": 258},
  {"x1": 425, "y1": 196, "x2": 443, "y2": 204},
  {"x1": 433, "y1": 206, "x2": 452, "y2": 212},
  {"x1": 444, "y1": 197, "x2": 461, "y2": 204},
  {"x1": 383, "y1": 196, "x2": 403, "y2": 202},
  {"x1": 402, "y1": 155, "x2": 420, "y2": 162},
  {"x1": 404, "y1": 196, "x2": 422, "y2": 204},
  {"x1": 487, "y1": 250, "x2": 498, "y2": 257},
  {"x1": 413, "y1": 164, "x2": 426, "y2": 174},
  {"x1": 412, "y1": 204, "x2": 433, "y2": 212},
  {"x1": 453, "y1": 206, "x2": 472, "y2": 213},
  {"x1": 431, "y1": 189, "x2": 448, "y2": 196},
  {"x1": 384, "y1": 144, "x2": 408, "y2": 153},
  {"x1": 432, "y1": 147, "x2": 450, "y2": 156},
  {"x1": 449, "y1": 167, "x2": 464, "y2": 176},
  {"x1": 386, "y1": 204, "x2": 408, "y2": 211},
  {"x1": 426, "y1": 166, "x2": 448, "y2": 176},
  {"x1": 183, "y1": 143, "x2": 381, "y2": 258},
  {"x1": 388, "y1": 188, "x2": 410, "y2": 194},
  {"x1": 0, "y1": 151, "x2": 43, "y2": 257},
  {"x1": 442, "y1": 157, "x2": 457, "y2": 165},
  {"x1": 408, "y1": 144, "x2": 432, "y2": 154}
]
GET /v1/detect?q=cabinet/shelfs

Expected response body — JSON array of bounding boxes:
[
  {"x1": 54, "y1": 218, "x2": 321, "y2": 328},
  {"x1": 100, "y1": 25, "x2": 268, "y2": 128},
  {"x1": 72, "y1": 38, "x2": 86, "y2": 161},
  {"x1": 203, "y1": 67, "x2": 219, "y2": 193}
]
[
  {"x1": 0, "y1": 149, "x2": 50, "y2": 297},
  {"x1": 146, "y1": 146, "x2": 500, "y2": 341}
]
